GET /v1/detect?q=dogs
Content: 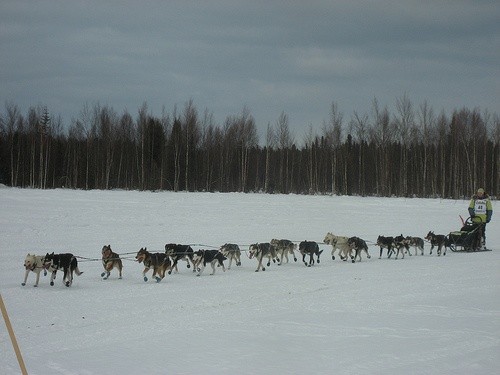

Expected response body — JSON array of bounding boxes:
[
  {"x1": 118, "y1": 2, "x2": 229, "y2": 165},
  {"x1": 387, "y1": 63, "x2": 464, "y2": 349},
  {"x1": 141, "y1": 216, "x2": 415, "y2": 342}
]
[
  {"x1": 425, "y1": 231, "x2": 449, "y2": 256},
  {"x1": 271, "y1": 239, "x2": 298, "y2": 262},
  {"x1": 101, "y1": 244, "x2": 122, "y2": 280},
  {"x1": 136, "y1": 247, "x2": 172, "y2": 283},
  {"x1": 299, "y1": 240, "x2": 324, "y2": 267},
  {"x1": 248, "y1": 243, "x2": 281, "y2": 272},
  {"x1": 377, "y1": 233, "x2": 424, "y2": 259},
  {"x1": 324, "y1": 231, "x2": 371, "y2": 263},
  {"x1": 22, "y1": 252, "x2": 85, "y2": 287},
  {"x1": 164, "y1": 244, "x2": 199, "y2": 274},
  {"x1": 220, "y1": 243, "x2": 241, "y2": 269},
  {"x1": 192, "y1": 249, "x2": 227, "y2": 276}
]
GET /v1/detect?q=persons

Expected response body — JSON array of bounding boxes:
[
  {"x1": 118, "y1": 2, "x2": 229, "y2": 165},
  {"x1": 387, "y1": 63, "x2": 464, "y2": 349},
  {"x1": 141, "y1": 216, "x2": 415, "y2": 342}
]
[{"x1": 468, "y1": 188, "x2": 493, "y2": 248}]
